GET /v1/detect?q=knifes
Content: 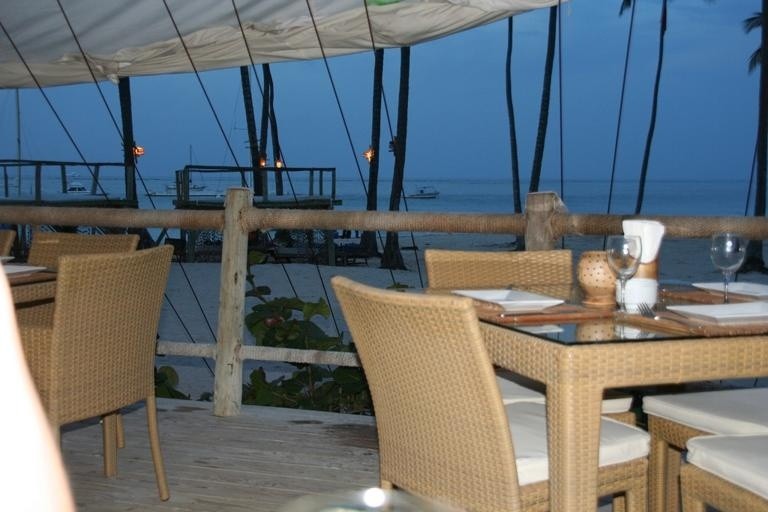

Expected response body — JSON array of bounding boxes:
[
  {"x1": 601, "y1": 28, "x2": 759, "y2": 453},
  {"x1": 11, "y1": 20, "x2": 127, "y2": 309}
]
[{"x1": 496, "y1": 308, "x2": 583, "y2": 318}]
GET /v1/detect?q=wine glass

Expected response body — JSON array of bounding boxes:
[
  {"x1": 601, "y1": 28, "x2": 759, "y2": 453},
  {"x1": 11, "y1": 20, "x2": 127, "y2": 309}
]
[
  {"x1": 605, "y1": 233, "x2": 641, "y2": 313},
  {"x1": 711, "y1": 232, "x2": 748, "y2": 305}
]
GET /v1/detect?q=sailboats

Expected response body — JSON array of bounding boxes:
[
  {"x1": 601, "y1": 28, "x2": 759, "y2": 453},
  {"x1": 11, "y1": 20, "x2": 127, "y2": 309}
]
[{"x1": 143, "y1": 146, "x2": 227, "y2": 197}]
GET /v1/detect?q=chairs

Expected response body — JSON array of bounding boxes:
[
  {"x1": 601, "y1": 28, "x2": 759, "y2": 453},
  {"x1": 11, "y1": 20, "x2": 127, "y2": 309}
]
[
  {"x1": 423, "y1": 248, "x2": 638, "y2": 427},
  {"x1": 14, "y1": 242, "x2": 175, "y2": 502},
  {"x1": 330, "y1": 274, "x2": 653, "y2": 511},
  {"x1": 25, "y1": 232, "x2": 141, "y2": 447},
  {"x1": 0, "y1": 228, "x2": 18, "y2": 265}
]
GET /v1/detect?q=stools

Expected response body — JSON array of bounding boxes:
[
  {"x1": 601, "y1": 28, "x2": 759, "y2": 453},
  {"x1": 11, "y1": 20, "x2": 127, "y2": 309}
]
[
  {"x1": 678, "y1": 434, "x2": 768, "y2": 512},
  {"x1": 641, "y1": 386, "x2": 768, "y2": 512}
]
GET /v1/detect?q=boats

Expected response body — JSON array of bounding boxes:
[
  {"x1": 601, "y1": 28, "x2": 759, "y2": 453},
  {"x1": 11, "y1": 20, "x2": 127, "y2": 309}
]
[
  {"x1": 59, "y1": 180, "x2": 111, "y2": 197},
  {"x1": 399, "y1": 183, "x2": 440, "y2": 200}
]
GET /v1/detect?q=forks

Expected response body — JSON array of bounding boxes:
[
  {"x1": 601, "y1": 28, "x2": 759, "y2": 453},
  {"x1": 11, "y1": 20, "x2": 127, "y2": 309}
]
[{"x1": 636, "y1": 302, "x2": 705, "y2": 332}]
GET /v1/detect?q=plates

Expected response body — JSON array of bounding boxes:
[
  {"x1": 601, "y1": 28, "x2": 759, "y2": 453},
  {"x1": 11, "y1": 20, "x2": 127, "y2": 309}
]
[
  {"x1": 1, "y1": 263, "x2": 47, "y2": 279},
  {"x1": 0, "y1": 255, "x2": 16, "y2": 266},
  {"x1": 665, "y1": 298, "x2": 767, "y2": 325},
  {"x1": 450, "y1": 288, "x2": 564, "y2": 313},
  {"x1": 687, "y1": 280, "x2": 766, "y2": 298}
]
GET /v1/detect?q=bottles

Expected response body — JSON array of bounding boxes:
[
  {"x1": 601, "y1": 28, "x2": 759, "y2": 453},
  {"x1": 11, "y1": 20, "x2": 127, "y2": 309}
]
[{"x1": 623, "y1": 242, "x2": 659, "y2": 280}]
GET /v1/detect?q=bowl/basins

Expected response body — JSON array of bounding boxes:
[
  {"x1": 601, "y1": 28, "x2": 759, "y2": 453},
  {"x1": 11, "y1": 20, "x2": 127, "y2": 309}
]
[{"x1": 614, "y1": 279, "x2": 660, "y2": 315}]
